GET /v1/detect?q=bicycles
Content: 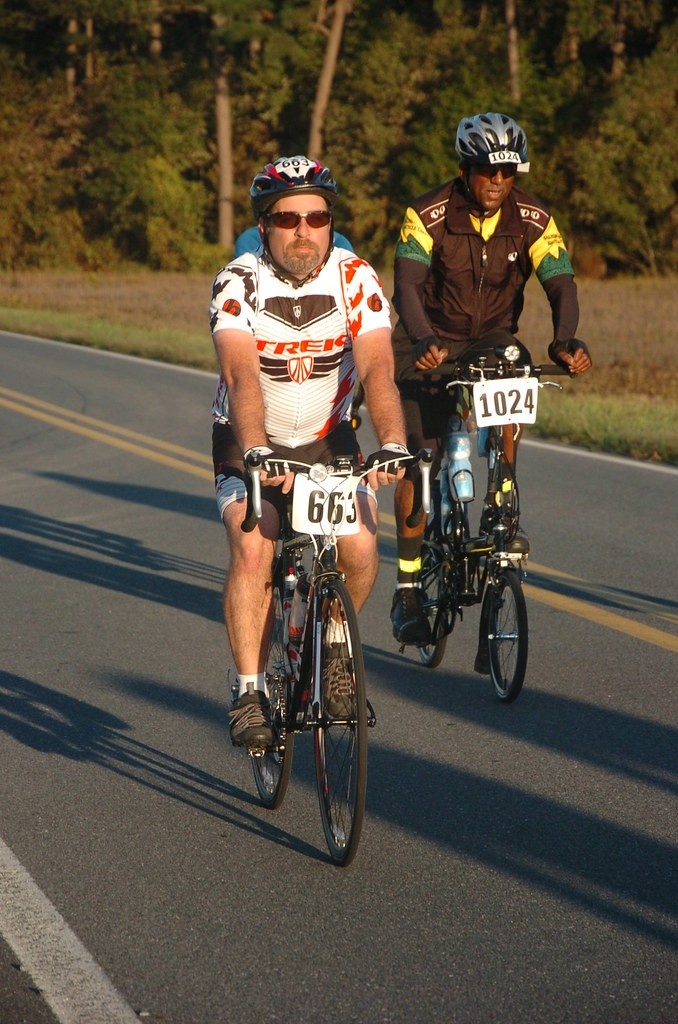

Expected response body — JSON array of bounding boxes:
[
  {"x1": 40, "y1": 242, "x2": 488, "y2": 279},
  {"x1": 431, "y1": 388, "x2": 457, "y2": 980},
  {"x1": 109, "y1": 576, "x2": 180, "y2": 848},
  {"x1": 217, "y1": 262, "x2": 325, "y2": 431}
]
[
  {"x1": 241, "y1": 450, "x2": 437, "y2": 866},
  {"x1": 412, "y1": 348, "x2": 576, "y2": 704}
]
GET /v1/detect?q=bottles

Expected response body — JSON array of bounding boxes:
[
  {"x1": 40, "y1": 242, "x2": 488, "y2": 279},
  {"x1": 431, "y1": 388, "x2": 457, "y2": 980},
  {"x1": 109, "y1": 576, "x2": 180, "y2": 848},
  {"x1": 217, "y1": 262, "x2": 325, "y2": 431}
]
[
  {"x1": 283, "y1": 565, "x2": 312, "y2": 643},
  {"x1": 446, "y1": 431, "x2": 474, "y2": 502}
]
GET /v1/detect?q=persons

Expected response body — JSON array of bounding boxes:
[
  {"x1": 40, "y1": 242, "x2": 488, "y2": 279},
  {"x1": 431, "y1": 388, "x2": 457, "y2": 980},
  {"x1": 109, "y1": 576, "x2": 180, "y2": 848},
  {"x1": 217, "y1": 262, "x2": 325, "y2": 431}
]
[
  {"x1": 390, "y1": 112, "x2": 591, "y2": 645},
  {"x1": 234, "y1": 225, "x2": 354, "y2": 261},
  {"x1": 207, "y1": 155, "x2": 411, "y2": 749}
]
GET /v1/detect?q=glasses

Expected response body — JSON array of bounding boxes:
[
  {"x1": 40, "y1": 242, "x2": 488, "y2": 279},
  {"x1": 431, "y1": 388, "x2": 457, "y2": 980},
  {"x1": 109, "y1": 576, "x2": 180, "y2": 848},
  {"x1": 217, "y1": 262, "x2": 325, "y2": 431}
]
[
  {"x1": 259, "y1": 211, "x2": 333, "y2": 229},
  {"x1": 469, "y1": 159, "x2": 519, "y2": 178}
]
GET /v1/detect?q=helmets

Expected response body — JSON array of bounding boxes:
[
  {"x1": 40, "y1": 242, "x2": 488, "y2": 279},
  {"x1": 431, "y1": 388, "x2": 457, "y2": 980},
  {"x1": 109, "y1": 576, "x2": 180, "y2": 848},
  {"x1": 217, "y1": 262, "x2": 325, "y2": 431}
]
[
  {"x1": 249, "y1": 155, "x2": 339, "y2": 223},
  {"x1": 455, "y1": 112, "x2": 528, "y2": 164}
]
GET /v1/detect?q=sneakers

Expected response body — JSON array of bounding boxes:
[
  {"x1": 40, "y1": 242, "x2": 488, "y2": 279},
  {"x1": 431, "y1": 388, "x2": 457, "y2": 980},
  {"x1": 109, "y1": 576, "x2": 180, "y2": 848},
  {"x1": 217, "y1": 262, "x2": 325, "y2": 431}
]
[
  {"x1": 390, "y1": 588, "x2": 432, "y2": 647},
  {"x1": 478, "y1": 507, "x2": 530, "y2": 554},
  {"x1": 228, "y1": 682, "x2": 276, "y2": 749},
  {"x1": 321, "y1": 633, "x2": 357, "y2": 721}
]
